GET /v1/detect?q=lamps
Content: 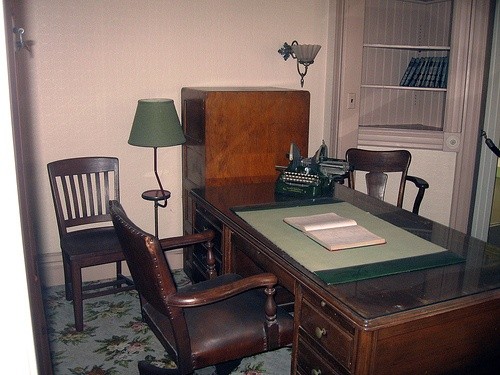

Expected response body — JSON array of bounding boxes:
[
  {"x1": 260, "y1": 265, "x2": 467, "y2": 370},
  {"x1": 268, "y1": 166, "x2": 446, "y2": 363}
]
[
  {"x1": 128, "y1": 98, "x2": 185, "y2": 238},
  {"x1": 278, "y1": 40, "x2": 321, "y2": 88}
]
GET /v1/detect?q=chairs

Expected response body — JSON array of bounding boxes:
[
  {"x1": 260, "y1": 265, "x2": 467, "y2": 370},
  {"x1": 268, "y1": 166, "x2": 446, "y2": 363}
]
[
  {"x1": 47, "y1": 157, "x2": 143, "y2": 331},
  {"x1": 340, "y1": 148, "x2": 429, "y2": 215},
  {"x1": 108, "y1": 199, "x2": 294, "y2": 375}
]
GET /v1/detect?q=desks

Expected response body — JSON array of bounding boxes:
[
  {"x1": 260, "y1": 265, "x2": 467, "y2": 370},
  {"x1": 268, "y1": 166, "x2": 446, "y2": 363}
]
[{"x1": 190, "y1": 181, "x2": 500, "y2": 375}]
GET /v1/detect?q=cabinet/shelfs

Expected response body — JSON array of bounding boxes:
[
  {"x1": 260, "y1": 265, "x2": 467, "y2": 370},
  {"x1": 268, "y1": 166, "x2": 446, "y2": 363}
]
[
  {"x1": 192, "y1": 210, "x2": 266, "y2": 277},
  {"x1": 181, "y1": 87, "x2": 311, "y2": 283},
  {"x1": 296, "y1": 296, "x2": 500, "y2": 375},
  {"x1": 329, "y1": 0, "x2": 476, "y2": 229}
]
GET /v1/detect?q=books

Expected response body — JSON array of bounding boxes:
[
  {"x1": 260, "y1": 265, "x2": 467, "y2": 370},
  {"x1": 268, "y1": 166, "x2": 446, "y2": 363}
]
[
  {"x1": 399, "y1": 56, "x2": 448, "y2": 88},
  {"x1": 283, "y1": 212, "x2": 386, "y2": 251}
]
